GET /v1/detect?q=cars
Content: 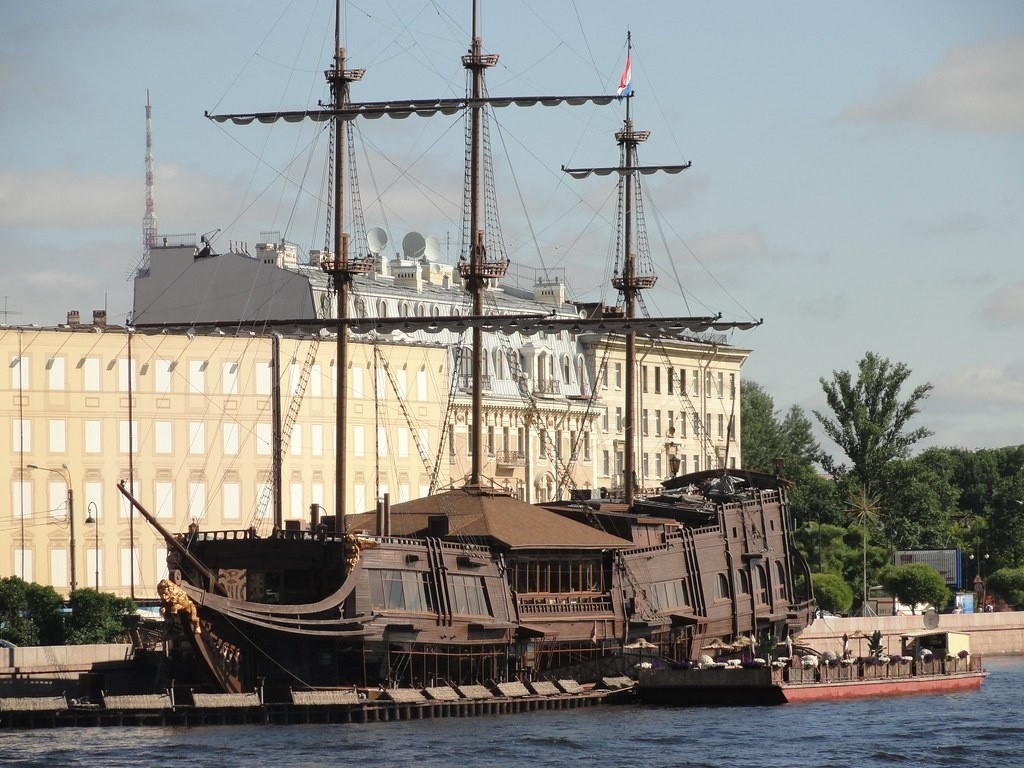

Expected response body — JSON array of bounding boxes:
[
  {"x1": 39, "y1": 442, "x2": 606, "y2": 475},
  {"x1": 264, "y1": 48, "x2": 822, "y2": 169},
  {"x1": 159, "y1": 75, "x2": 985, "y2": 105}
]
[{"x1": 815, "y1": 609, "x2": 840, "y2": 619}]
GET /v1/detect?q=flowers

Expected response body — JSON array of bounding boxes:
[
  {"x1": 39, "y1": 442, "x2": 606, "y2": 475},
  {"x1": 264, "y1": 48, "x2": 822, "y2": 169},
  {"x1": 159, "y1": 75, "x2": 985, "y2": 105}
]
[
  {"x1": 863, "y1": 654, "x2": 914, "y2": 664},
  {"x1": 635, "y1": 654, "x2": 819, "y2": 671},
  {"x1": 944, "y1": 649, "x2": 969, "y2": 660},
  {"x1": 819, "y1": 650, "x2": 859, "y2": 668},
  {"x1": 919, "y1": 647, "x2": 937, "y2": 662}
]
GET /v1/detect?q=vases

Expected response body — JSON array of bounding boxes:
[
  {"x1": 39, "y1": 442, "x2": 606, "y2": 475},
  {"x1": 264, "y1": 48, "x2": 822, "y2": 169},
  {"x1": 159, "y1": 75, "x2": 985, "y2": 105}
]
[
  {"x1": 914, "y1": 658, "x2": 943, "y2": 675},
  {"x1": 638, "y1": 665, "x2": 818, "y2": 684},
  {"x1": 861, "y1": 662, "x2": 913, "y2": 678},
  {"x1": 943, "y1": 658, "x2": 970, "y2": 673},
  {"x1": 818, "y1": 664, "x2": 860, "y2": 680}
]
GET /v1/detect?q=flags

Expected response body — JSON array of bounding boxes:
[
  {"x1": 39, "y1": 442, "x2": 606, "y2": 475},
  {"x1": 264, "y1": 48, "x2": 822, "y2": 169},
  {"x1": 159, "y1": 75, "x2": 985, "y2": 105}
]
[{"x1": 618, "y1": 57, "x2": 631, "y2": 101}]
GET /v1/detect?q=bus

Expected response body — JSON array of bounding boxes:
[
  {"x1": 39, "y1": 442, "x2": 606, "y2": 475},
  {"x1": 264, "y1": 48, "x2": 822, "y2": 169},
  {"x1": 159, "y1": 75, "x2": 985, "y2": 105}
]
[{"x1": 868, "y1": 584, "x2": 938, "y2": 617}]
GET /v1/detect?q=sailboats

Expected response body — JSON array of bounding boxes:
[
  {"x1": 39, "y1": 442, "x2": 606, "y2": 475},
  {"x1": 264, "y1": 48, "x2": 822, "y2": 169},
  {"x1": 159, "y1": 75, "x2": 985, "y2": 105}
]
[{"x1": 117, "y1": 0, "x2": 822, "y2": 719}]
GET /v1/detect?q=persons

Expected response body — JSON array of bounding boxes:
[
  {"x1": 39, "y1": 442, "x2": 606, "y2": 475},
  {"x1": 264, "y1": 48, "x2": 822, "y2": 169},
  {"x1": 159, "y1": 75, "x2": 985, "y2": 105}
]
[
  {"x1": 976, "y1": 605, "x2": 983, "y2": 613},
  {"x1": 895, "y1": 610, "x2": 900, "y2": 616},
  {"x1": 951, "y1": 607, "x2": 961, "y2": 615},
  {"x1": 985, "y1": 602, "x2": 993, "y2": 613}
]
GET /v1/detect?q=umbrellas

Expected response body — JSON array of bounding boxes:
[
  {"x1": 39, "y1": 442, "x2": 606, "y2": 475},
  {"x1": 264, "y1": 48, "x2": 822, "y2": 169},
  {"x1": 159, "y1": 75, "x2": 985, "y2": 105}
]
[
  {"x1": 749, "y1": 634, "x2": 756, "y2": 657},
  {"x1": 730, "y1": 635, "x2": 758, "y2": 663},
  {"x1": 701, "y1": 638, "x2": 734, "y2": 663},
  {"x1": 785, "y1": 635, "x2": 792, "y2": 657},
  {"x1": 623, "y1": 637, "x2": 658, "y2": 667}
]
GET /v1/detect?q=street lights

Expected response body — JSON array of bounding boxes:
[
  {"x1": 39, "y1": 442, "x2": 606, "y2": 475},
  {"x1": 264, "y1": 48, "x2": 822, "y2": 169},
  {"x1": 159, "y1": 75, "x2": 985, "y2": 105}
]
[
  {"x1": 84, "y1": 501, "x2": 100, "y2": 593},
  {"x1": 876, "y1": 509, "x2": 899, "y2": 615},
  {"x1": 26, "y1": 462, "x2": 79, "y2": 592},
  {"x1": 968, "y1": 537, "x2": 990, "y2": 609}
]
